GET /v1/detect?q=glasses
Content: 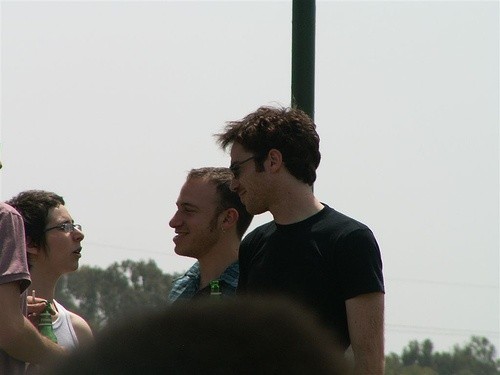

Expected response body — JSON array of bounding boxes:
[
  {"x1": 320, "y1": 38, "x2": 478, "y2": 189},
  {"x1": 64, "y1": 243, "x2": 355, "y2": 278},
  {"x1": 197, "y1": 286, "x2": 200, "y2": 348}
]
[
  {"x1": 43, "y1": 222, "x2": 82, "y2": 233},
  {"x1": 230, "y1": 155, "x2": 255, "y2": 178}
]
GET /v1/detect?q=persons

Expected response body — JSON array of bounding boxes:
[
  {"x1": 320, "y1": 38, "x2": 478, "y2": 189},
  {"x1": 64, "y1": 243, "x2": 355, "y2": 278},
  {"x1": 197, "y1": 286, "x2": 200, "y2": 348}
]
[
  {"x1": 3, "y1": 189, "x2": 97, "y2": 375},
  {"x1": 213, "y1": 103, "x2": 385, "y2": 375},
  {"x1": 166, "y1": 167, "x2": 254, "y2": 317},
  {"x1": 46, "y1": 294, "x2": 357, "y2": 375},
  {"x1": 0, "y1": 202, "x2": 66, "y2": 375}
]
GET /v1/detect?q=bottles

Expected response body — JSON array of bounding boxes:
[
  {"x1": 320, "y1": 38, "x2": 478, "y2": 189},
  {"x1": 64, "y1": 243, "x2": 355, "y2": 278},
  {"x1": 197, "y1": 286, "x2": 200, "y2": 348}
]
[
  {"x1": 211, "y1": 280, "x2": 224, "y2": 305},
  {"x1": 39, "y1": 301, "x2": 57, "y2": 344}
]
